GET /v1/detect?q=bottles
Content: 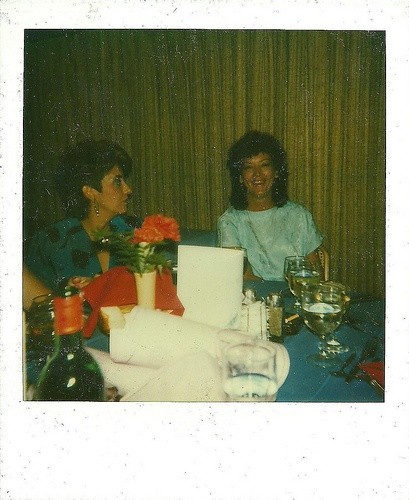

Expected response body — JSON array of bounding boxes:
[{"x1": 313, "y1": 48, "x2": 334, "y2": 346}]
[{"x1": 34, "y1": 286, "x2": 104, "y2": 401}]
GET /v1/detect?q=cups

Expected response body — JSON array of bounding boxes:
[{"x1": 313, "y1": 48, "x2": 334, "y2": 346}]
[
  {"x1": 221, "y1": 343, "x2": 277, "y2": 401},
  {"x1": 226, "y1": 247, "x2": 248, "y2": 276}
]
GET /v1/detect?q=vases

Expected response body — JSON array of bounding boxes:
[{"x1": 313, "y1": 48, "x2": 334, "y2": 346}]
[{"x1": 134, "y1": 270, "x2": 157, "y2": 309}]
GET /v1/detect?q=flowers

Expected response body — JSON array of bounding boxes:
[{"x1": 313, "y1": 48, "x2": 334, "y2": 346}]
[{"x1": 92, "y1": 214, "x2": 180, "y2": 276}]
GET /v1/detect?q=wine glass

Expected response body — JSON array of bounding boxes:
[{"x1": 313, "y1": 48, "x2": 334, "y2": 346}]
[{"x1": 283, "y1": 256, "x2": 349, "y2": 368}]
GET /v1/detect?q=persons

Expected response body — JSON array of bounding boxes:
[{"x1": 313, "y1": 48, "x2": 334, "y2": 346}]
[
  {"x1": 22, "y1": 138, "x2": 139, "y2": 335},
  {"x1": 216, "y1": 130, "x2": 326, "y2": 283}
]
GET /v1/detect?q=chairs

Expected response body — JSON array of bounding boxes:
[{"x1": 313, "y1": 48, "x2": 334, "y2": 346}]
[{"x1": 318, "y1": 245, "x2": 329, "y2": 281}]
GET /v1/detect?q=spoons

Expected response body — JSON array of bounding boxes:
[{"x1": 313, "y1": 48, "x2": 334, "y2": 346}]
[{"x1": 329, "y1": 353, "x2": 356, "y2": 378}]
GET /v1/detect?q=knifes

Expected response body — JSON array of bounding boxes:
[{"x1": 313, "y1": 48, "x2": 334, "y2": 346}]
[{"x1": 346, "y1": 339, "x2": 372, "y2": 384}]
